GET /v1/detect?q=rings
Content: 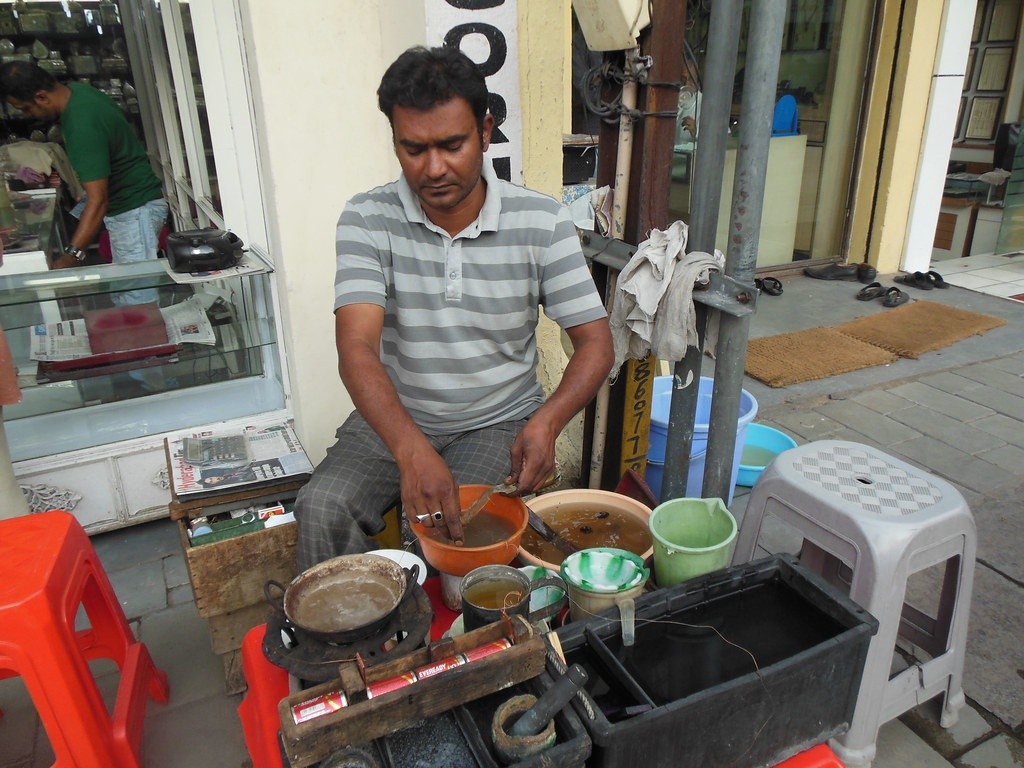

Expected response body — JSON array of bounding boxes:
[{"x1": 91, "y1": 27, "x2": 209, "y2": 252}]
[
  {"x1": 416, "y1": 512, "x2": 430, "y2": 523},
  {"x1": 430, "y1": 511, "x2": 442, "y2": 522}
]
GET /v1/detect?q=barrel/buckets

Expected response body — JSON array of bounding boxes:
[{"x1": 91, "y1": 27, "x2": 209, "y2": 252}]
[{"x1": 645, "y1": 375, "x2": 758, "y2": 510}]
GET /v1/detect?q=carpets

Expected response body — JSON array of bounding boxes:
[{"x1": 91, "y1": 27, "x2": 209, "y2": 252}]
[
  {"x1": 739, "y1": 324, "x2": 900, "y2": 390},
  {"x1": 838, "y1": 299, "x2": 1007, "y2": 357}
]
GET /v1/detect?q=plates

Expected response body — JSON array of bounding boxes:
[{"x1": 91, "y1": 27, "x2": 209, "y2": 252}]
[{"x1": 364, "y1": 549, "x2": 428, "y2": 586}]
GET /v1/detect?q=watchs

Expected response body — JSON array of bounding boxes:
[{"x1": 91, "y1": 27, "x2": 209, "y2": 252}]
[{"x1": 63, "y1": 245, "x2": 87, "y2": 263}]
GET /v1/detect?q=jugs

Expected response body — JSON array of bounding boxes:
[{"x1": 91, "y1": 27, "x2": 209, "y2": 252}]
[
  {"x1": 648, "y1": 496, "x2": 739, "y2": 588},
  {"x1": 560, "y1": 547, "x2": 651, "y2": 647},
  {"x1": 458, "y1": 564, "x2": 568, "y2": 636}
]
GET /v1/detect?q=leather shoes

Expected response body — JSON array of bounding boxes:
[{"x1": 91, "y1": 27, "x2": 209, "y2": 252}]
[
  {"x1": 804, "y1": 264, "x2": 858, "y2": 282},
  {"x1": 851, "y1": 262, "x2": 876, "y2": 284}
]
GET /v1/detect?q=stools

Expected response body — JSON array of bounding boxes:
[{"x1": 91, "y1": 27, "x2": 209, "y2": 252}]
[
  {"x1": 1, "y1": 504, "x2": 168, "y2": 767},
  {"x1": 730, "y1": 441, "x2": 976, "y2": 765},
  {"x1": 238, "y1": 576, "x2": 460, "y2": 768}
]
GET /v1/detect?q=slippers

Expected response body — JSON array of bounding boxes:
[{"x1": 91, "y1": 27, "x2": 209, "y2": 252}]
[
  {"x1": 884, "y1": 286, "x2": 909, "y2": 308},
  {"x1": 754, "y1": 278, "x2": 763, "y2": 295},
  {"x1": 925, "y1": 270, "x2": 949, "y2": 289},
  {"x1": 893, "y1": 271, "x2": 934, "y2": 290},
  {"x1": 856, "y1": 282, "x2": 889, "y2": 301},
  {"x1": 762, "y1": 276, "x2": 783, "y2": 296}
]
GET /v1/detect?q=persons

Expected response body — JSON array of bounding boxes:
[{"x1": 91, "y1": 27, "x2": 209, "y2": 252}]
[
  {"x1": 292, "y1": 42, "x2": 618, "y2": 594},
  {"x1": 1, "y1": 59, "x2": 179, "y2": 390},
  {"x1": 674, "y1": 59, "x2": 732, "y2": 147},
  {"x1": 1, "y1": 229, "x2": 34, "y2": 520}
]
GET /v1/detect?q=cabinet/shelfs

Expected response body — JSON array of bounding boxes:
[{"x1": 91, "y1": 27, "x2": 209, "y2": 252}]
[{"x1": 164, "y1": 423, "x2": 317, "y2": 696}]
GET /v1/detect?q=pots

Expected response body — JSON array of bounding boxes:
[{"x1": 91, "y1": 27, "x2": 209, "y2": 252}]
[{"x1": 262, "y1": 553, "x2": 420, "y2": 644}]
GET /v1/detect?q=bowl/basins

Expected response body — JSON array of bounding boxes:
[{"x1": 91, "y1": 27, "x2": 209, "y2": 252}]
[
  {"x1": 734, "y1": 422, "x2": 799, "y2": 487},
  {"x1": 516, "y1": 488, "x2": 655, "y2": 573},
  {"x1": 409, "y1": 484, "x2": 530, "y2": 576}
]
